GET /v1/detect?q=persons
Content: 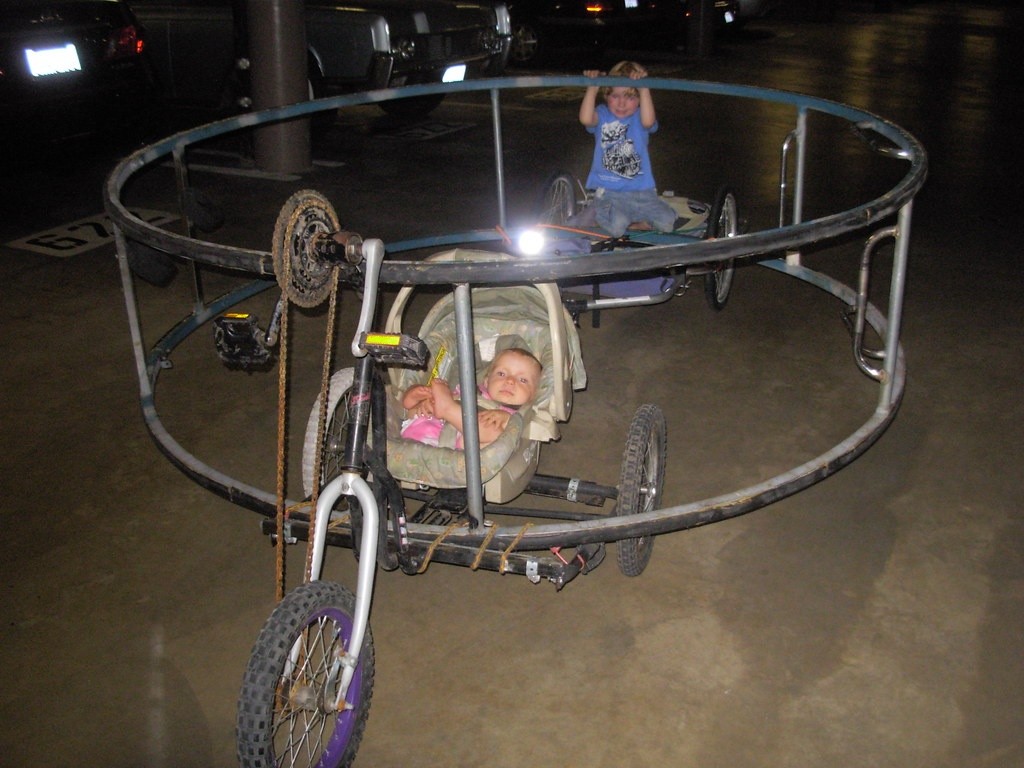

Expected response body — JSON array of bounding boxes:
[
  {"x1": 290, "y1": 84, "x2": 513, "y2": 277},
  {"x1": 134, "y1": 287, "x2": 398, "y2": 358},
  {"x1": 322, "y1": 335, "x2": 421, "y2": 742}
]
[
  {"x1": 579, "y1": 61, "x2": 679, "y2": 239},
  {"x1": 399, "y1": 348, "x2": 542, "y2": 449}
]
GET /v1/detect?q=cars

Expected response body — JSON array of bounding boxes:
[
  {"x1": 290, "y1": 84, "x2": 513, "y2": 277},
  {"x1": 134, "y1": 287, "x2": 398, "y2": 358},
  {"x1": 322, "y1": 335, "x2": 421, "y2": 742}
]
[{"x1": 0, "y1": 0, "x2": 513, "y2": 225}]
